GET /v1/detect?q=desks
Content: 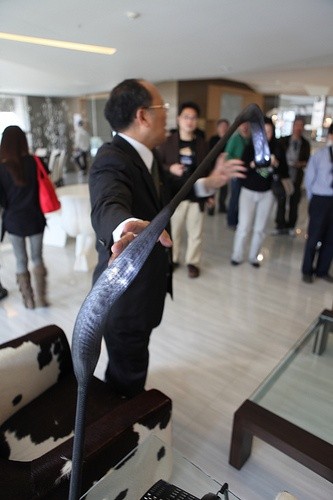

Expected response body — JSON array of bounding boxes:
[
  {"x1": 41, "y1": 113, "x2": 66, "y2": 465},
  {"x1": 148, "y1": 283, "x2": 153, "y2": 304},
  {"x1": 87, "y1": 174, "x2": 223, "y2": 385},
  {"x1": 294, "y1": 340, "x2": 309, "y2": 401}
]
[{"x1": 43, "y1": 183, "x2": 100, "y2": 273}]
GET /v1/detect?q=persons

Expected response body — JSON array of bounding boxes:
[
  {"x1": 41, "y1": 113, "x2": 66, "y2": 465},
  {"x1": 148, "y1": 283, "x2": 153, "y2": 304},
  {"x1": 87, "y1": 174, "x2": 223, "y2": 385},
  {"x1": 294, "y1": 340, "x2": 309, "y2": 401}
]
[
  {"x1": 0, "y1": 125, "x2": 51, "y2": 310},
  {"x1": 48, "y1": 119, "x2": 88, "y2": 175},
  {"x1": 89, "y1": 78, "x2": 247, "y2": 390},
  {"x1": 155, "y1": 102, "x2": 333, "y2": 285}
]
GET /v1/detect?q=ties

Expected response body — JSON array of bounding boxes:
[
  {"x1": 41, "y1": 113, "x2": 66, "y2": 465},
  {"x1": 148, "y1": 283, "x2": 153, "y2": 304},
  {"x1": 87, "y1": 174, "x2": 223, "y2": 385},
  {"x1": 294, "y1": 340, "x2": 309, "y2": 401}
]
[
  {"x1": 293, "y1": 141, "x2": 297, "y2": 151},
  {"x1": 329, "y1": 146, "x2": 333, "y2": 191},
  {"x1": 151, "y1": 159, "x2": 160, "y2": 192}
]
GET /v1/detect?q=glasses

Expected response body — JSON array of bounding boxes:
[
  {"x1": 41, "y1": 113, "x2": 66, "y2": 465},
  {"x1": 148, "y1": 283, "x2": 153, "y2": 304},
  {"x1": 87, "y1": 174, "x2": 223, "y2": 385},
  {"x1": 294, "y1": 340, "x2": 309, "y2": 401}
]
[{"x1": 134, "y1": 102, "x2": 170, "y2": 120}]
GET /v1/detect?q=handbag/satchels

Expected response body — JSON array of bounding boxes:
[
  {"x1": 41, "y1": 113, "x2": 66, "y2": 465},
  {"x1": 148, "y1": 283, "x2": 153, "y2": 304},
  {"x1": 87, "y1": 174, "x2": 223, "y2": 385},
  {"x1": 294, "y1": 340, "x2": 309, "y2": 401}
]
[{"x1": 31, "y1": 154, "x2": 61, "y2": 214}]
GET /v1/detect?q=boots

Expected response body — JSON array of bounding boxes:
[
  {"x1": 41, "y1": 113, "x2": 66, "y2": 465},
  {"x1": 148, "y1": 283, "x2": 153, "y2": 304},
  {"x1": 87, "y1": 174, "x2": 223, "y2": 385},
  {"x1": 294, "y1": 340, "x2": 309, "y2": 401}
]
[
  {"x1": 17, "y1": 271, "x2": 36, "y2": 308},
  {"x1": 33, "y1": 266, "x2": 50, "y2": 307}
]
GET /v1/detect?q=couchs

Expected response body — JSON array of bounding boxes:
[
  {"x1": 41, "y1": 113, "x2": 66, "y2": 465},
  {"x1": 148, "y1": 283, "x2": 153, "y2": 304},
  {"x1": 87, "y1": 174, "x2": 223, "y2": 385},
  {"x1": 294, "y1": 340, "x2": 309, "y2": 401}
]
[{"x1": 0, "y1": 323, "x2": 173, "y2": 500}]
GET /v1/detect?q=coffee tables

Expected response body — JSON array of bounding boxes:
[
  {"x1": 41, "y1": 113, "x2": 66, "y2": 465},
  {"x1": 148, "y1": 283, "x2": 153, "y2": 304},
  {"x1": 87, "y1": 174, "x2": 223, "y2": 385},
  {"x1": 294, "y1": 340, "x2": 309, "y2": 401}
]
[{"x1": 227, "y1": 307, "x2": 333, "y2": 485}]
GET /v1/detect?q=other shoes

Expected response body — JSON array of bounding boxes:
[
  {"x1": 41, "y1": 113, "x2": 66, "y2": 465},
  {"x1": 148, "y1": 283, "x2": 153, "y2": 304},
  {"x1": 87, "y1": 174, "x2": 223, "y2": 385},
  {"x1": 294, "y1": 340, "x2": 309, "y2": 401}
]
[
  {"x1": 288, "y1": 228, "x2": 296, "y2": 236},
  {"x1": 231, "y1": 259, "x2": 238, "y2": 266},
  {"x1": 187, "y1": 264, "x2": 199, "y2": 278},
  {"x1": 0, "y1": 289, "x2": 8, "y2": 298},
  {"x1": 315, "y1": 272, "x2": 333, "y2": 283},
  {"x1": 302, "y1": 273, "x2": 312, "y2": 283},
  {"x1": 173, "y1": 262, "x2": 179, "y2": 268},
  {"x1": 252, "y1": 263, "x2": 260, "y2": 267},
  {"x1": 272, "y1": 229, "x2": 288, "y2": 235}
]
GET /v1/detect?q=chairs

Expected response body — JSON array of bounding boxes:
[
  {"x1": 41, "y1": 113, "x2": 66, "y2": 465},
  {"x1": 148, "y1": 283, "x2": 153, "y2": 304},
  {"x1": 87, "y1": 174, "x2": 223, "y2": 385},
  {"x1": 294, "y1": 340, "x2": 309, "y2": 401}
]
[{"x1": 79, "y1": 432, "x2": 301, "y2": 500}]
